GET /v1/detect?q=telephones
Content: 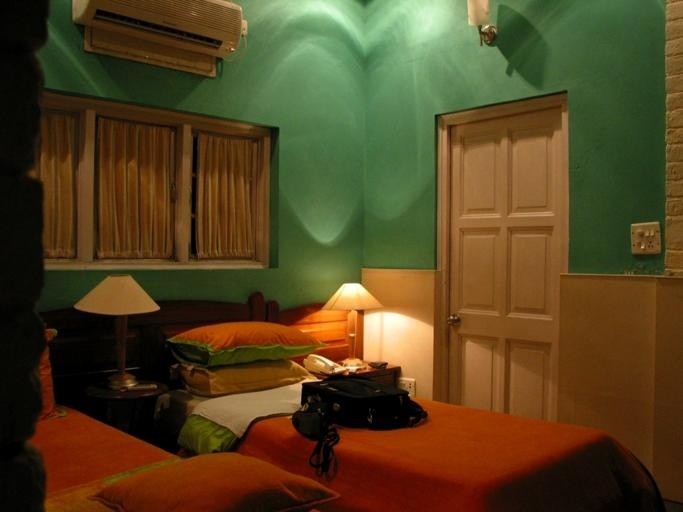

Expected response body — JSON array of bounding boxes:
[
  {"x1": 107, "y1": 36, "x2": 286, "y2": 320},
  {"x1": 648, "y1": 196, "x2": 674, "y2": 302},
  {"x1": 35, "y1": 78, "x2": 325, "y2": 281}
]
[{"x1": 302, "y1": 354, "x2": 350, "y2": 375}]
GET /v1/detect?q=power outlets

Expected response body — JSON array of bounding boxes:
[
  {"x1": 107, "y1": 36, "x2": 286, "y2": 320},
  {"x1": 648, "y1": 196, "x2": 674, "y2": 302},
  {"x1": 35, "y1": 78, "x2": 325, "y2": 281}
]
[{"x1": 396, "y1": 377, "x2": 415, "y2": 397}]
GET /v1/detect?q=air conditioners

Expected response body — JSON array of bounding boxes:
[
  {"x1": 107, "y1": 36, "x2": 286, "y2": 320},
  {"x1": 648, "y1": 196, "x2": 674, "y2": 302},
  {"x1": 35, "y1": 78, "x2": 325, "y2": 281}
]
[{"x1": 71, "y1": 1, "x2": 242, "y2": 58}]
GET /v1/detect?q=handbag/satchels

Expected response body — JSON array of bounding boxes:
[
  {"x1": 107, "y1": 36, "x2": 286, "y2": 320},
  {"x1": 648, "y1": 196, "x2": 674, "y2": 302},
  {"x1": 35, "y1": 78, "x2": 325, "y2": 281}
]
[{"x1": 300, "y1": 372, "x2": 427, "y2": 430}]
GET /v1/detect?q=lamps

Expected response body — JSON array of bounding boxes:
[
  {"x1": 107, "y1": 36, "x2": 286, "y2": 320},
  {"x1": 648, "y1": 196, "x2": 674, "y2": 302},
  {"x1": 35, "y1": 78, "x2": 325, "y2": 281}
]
[
  {"x1": 320, "y1": 282, "x2": 384, "y2": 369},
  {"x1": 467, "y1": 0, "x2": 496, "y2": 46},
  {"x1": 73, "y1": 272, "x2": 160, "y2": 390}
]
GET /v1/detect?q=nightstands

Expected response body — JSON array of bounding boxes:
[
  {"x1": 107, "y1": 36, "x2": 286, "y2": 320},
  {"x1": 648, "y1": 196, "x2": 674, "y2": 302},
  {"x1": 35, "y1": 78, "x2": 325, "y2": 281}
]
[{"x1": 310, "y1": 361, "x2": 400, "y2": 390}]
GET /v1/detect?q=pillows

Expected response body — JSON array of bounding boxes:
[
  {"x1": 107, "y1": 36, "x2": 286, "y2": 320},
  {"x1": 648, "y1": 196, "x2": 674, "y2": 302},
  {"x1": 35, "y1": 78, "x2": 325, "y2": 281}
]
[
  {"x1": 164, "y1": 320, "x2": 326, "y2": 399},
  {"x1": 84, "y1": 452, "x2": 341, "y2": 511}
]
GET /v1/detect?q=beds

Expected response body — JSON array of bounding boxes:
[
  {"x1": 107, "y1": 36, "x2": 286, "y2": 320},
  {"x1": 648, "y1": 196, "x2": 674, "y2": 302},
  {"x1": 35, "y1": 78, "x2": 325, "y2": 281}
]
[
  {"x1": 27, "y1": 404, "x2": 320, "y2": 512},
  {"x1": 164, "y1": 373, "x2": 614, "y2": 509}
]
